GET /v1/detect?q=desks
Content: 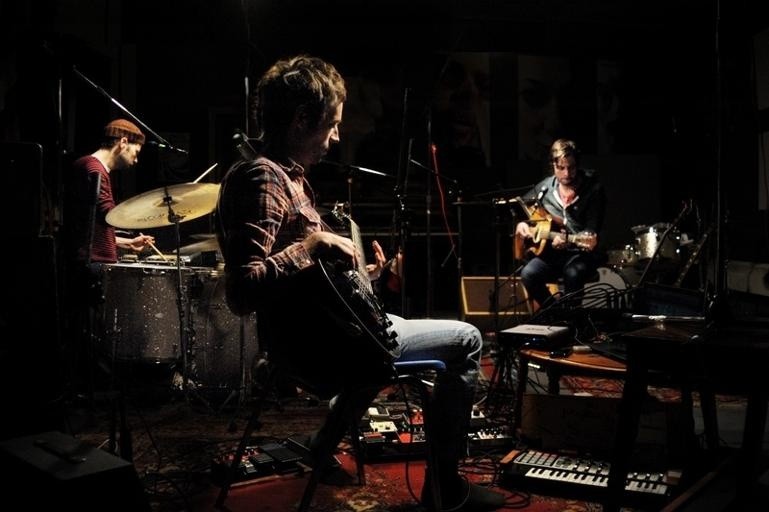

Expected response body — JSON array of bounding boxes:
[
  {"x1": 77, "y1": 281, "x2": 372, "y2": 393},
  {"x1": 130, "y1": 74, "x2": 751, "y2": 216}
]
[{"x1": 514, "y1": 347, "x2": 722, "y2": 511}]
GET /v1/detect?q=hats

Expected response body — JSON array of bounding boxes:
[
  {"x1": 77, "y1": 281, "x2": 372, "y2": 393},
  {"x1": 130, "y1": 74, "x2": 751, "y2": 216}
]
[{"x1": 107, "y1": 119, "x2": 145, "y2": 145}]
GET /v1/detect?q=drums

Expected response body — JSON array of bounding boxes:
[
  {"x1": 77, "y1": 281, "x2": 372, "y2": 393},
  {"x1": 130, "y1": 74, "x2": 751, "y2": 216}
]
[
  {"x1": 608, "y1": 248, "x2": 636, "y2": 268},
  {"x1": 194, "y1": 268, "x2": 262, "y2": 393},
  {"x1": 635, "y1": 224, "x2": 679, "y2": 263},
  {"x1": 98, "y1": 259, "x2": 190, "y2": 360}
]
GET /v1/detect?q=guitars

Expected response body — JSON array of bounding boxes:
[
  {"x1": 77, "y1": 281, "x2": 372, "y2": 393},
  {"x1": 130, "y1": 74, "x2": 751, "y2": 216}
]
[
  {"x1": 315, "y1": 201, "x2": 402, "y2": 361},
  {"x1": 513, "y1": 217, "x2": 595, "y2": 260}
]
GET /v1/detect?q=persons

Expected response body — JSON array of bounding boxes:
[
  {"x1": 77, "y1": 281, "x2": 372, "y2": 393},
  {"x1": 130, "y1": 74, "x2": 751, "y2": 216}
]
[
  {"x1": 219, "y1": 56, "x2": 506, "y2": 512},
  {"x1": 521, "y1": 138, "x2": 601, "y2": 324},
  {"x1": 63, "y1": 119, "x2": 156, "y2": 398}
]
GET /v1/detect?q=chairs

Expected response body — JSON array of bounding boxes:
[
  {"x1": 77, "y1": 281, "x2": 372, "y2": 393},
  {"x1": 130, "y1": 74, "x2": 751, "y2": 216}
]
[{"x1": 210, "y1": 215, "x2": 446, "y2": 512}]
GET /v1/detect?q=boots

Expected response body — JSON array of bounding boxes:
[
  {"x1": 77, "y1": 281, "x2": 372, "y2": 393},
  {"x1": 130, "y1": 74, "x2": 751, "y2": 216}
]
[{"x1": 422, "y1": 467, "x2": 505, "y2": 512}]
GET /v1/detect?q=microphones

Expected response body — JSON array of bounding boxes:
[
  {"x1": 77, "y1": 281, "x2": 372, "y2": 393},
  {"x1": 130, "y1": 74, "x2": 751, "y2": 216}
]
[
  {"x1": 534, "y1": 181, "x2": 551, "y2": 209},
  {"x1": 150, "y1": 138, "x2": 192, "y2": 155}
]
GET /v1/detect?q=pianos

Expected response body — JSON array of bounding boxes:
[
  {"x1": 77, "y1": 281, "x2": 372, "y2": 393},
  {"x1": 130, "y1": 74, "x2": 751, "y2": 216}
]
[{"x1": 505, "y1": 447, "x2": 669, "y2": 504}]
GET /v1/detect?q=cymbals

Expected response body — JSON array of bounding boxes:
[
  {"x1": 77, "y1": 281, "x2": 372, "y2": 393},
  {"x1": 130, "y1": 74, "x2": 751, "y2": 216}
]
[{"x1": 106, "y1": 183, "x2": 220, "y2": 228}]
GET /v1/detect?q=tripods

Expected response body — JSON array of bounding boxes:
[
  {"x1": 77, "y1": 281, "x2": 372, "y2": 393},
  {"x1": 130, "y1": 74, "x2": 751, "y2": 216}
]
[
  {"x1": 212, "y1": 314, "x2": 284, "y2": 433},
  {"x1": 161, "y1": 214, "x2": 215, "y2": 417}
]
